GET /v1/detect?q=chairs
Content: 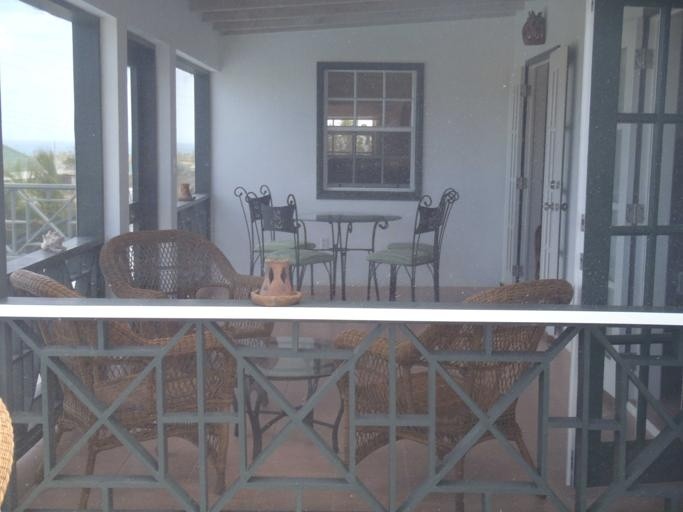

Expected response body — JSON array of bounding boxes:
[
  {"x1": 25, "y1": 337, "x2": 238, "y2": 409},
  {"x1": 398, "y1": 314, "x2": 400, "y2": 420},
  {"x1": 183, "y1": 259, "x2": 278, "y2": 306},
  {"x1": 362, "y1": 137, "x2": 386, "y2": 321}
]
[
  {"x1": 98, "y1": 229, "x2": 276, "y2": 456},
  {"x1": 233, "y1": 184, "x2": 314, "y2": 297},
  {"x1": 0, "y1": 394, "x2": 17, "y2": 511},
  {"x1": 244, "y1": 191, "x2": 335, "y2": 302},
  {"x1": 331, "y1": 278, "x2": 575, "y2": 512},
  {"x1": 8, "y1": 267, "x2": 244, "y2": 512},
  {"x1": 364, "y1": 187, "x2": 459, "y2": 303}
]
